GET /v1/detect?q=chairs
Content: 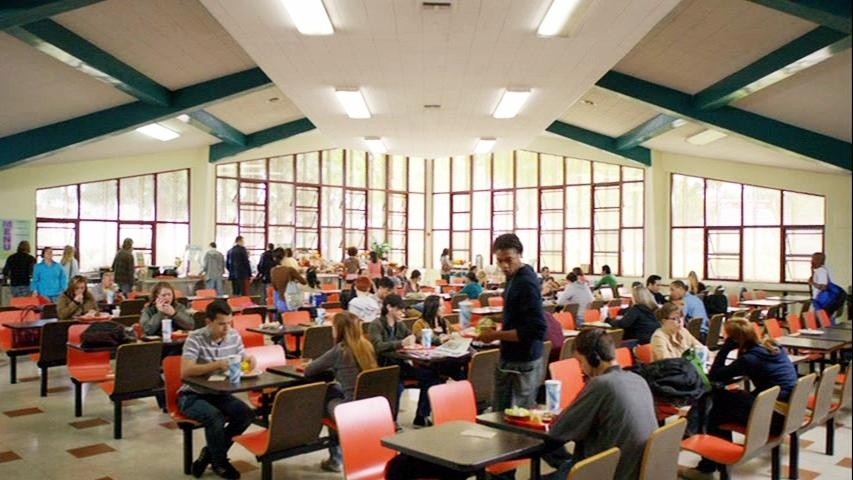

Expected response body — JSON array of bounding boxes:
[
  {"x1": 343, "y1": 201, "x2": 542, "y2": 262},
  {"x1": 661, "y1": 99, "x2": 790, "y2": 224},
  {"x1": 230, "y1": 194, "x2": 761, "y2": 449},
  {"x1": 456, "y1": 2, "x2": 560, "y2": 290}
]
[
  {"x1": 0, "y1": 289, "x2": 164, "y2": 440},
  {"x1": 468, "y1": 349, "x2": 500, "y2": 405},
  {"x1": 679, "y1": 385, "x2": 780, "y2": 479},
  {"x1": 805, "y1": 365, "x2": 852, "y2": 456},
  {"x1": 322, "y1": 365, "x2": 400, "y2": 435},
  {"x1": 637, "y1": 418, "x2": 687, "y2": 479},
  {"x1": 606, "y1": 328, "x2": 624, "y2": 349},
  {"x1": 542, "y1": 284, "x2": 633, "y2": 338},
  {"x1": 163, "y1": 355, "x2": 204, "y2": 476},
  {"x1": 687, "y1": 284, "x2": 853, "y2": 375},
  {"x1": 174, "y1": 279, "x2": 506, "y2": 363},
  {"x1": 548, "y1": 358, "x2": 585, "y2": 409},
  {"x1": 566, "y1": 447, "x2": 620, "y2": 480},
  {"x1": 788, "y1": 365, "x2": 839, "y2": 480},
  {"x1": 428, "y1": 380, "x2": 531, "y2": 473},
  {"x1": 720, "y1": 373, "x2": 814, "y2": 480},
  {"x1": 333, "y1": 396, "x2": 397, "y2": 480},
  {"x1": 243, "y1": 344, "x2": 287, "y2": 408},
  {"x1": 537, "y1": 340, "x2": 551, "y2": 388},
  {"x1": 232, "y1": 381, "x2": 328, "y2": 480},
  {"x1": 561, "y1": 336, "x2": 577, "y2": 360},
  {"x1": 398, "y1": 343, "x2": 468, "y2": 386},
  {"x1": 615, "y1": 343, "x2": 651, "y2": 368}
]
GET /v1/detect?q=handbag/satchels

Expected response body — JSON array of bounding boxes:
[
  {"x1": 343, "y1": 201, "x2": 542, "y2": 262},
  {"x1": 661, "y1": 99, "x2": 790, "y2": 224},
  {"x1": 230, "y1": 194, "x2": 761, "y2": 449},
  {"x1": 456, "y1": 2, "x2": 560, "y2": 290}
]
[
  {"x1": 623, "y1": 358, "x2": 704, "y2": 403},
  {"x1": 12, "y1": 327, "x2": 40, "y2": 347},
  {"x1": 79, "y1": 321, "x2": 136, "y2": 348},
  {"x1": 813, "y1": 283, "x2": 849, "y2": 318},
  {"x1": 703, "y1": 294, "x2": 727, "y2": 314},
  {"x1": 284, "y1": 281, "x2": 304, "y2": 311}
]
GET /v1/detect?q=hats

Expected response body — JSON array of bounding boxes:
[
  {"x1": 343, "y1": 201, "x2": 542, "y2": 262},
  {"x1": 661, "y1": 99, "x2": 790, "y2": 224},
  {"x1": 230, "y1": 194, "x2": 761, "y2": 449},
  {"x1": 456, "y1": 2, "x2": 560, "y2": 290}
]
[{"x1": 383, "y1": 294, "x2": 412, "y2": 309}]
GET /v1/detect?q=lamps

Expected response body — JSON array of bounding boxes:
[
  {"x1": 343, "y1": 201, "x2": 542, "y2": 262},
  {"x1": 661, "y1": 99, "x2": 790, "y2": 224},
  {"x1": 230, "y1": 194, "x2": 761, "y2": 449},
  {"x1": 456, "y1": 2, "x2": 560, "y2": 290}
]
[
  {"x1": 471, "y1": 138, "x2": 495, "y2": 156},
  {"x1": 335, "y1": 88, "x2": 373, "y2": 120},
  {"x1": 363, "y1": 137, "x2": 386, "y2": 156},
  {"x1": 138, "y1": 123, "x2": 180, "y2": 142},
  {"x1": 536, "y1": 1, "x2": 578, "y2": 37},
  {"x1": 280, "y1": 0, "x2": 335, "y2": 36},
  {"x1": 491, "y1": 88, "x2": 530, "y2": 121}
]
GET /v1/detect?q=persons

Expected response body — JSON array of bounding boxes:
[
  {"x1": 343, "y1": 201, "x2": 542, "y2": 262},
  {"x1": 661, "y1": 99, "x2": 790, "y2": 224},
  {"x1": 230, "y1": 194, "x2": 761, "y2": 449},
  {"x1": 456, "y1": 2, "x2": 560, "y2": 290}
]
[
  {"x1": 173, "y1": 294, "x2": 257, "y2": 480},
  {"x1": 604, "y1": 271, "x2": 708, "y2": 361},
  {"x1": 677, "y1": 318, "x2": 799, "y2": 480},
  {"x1": 300, "y1": 309, "x2": 379, "y2": 472},
  {"x1": 480, "y1": 234, "x2": 546, "y2": 412},
  {"x1": 140, "y1": 282, "x2": 195, "y2": 358},
  {"x1": 202, "y1": 235, "x2": 320, "y2": 320},
  {"x1": 3, "y1": 238, "x2": 133, "y2": 320},
  {"x1": 539, "y1": 266, "x2": 617, "y2": 324},
  {"x1": 808, "y1": 253, "x2": 829, "y2": 299},
  {"x1": 549, "y1": 325, "x2": 660, "y2": 479},
  {"x1": 341, "y1": 248, "x2": 481, "y2": 425}
]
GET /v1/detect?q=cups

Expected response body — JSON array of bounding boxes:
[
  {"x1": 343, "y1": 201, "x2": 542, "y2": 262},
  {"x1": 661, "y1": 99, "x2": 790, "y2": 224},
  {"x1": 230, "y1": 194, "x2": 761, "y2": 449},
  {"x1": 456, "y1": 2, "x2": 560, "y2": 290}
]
[
  {"x1": 161, "y1": 318, "x2": 172, "y2": 340},
  {"x1": 420, "y1": 328, "x2": 431, "y2": 348},
  {"x1": 105, "y1": 291, "x2": 113, "y2": 303},
  {"x1": 544, "y1": 379, "x2": 560, "y2": 413},
  {"x1": 304, "y1": 292, "x2": 309, "y2": 304},
  {"x1": 227, "y1": 354, "x2": 242, "y2": 385},
  {"x1": 458, "y1": 300, "x2": 472, "y2": 326},
  {"x1": 316, "y1": 308, "x2": 325, "y2": 326}
]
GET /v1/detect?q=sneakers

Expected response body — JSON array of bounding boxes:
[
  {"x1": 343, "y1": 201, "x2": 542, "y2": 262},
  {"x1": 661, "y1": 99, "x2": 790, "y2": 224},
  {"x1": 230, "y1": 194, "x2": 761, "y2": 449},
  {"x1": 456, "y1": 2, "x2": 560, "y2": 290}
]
[
  {"x1": 192, "y1": 447, "x2": 209, "y2": 478},
  {"x1": 212, "y1": 459, "x2": 240, "y2": 479},
  {"x1": 413, "y1": 417, "x2": 433, "y2": 429},
  {"x1": 321, "y1": 458, "x2": 342, "y2": 472},
  {"x1": 394, "y1": 420, "x2": 402, "y2": 433}
]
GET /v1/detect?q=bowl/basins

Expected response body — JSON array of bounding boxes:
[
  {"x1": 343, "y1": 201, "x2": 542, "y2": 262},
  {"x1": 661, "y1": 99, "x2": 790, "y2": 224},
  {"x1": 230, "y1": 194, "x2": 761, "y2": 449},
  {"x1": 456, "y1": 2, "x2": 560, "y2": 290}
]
[
  {"x1": 505, "y1": 412, "x2": 530, "y2": 419},
  {"x1": 111, "y1": 309, "x2": 120, "y2": 316}
]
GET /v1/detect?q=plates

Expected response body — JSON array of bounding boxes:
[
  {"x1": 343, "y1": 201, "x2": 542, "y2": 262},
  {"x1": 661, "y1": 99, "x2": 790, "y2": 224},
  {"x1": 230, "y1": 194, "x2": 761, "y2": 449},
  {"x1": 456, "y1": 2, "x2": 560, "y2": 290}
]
[
  {"x1": 223, "y1": 368, "x2": 262, "y2": 378},
  {"x1": 798, "y1": 328, "x2": 824, "y2": 336}
]
